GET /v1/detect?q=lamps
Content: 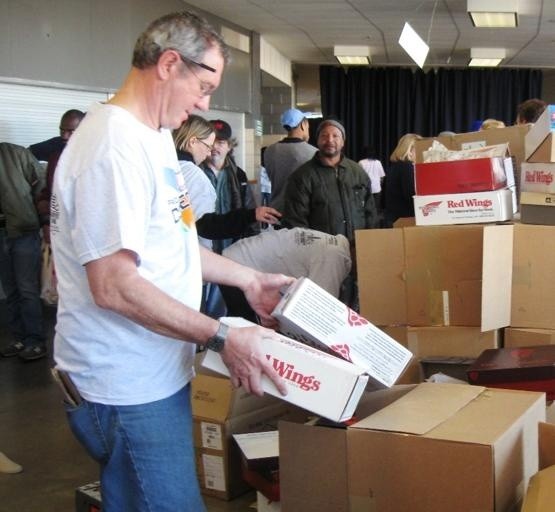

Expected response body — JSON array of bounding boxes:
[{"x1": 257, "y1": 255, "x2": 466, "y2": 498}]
[
  {"x1": 466, "y1": 47, "x2": 506, "y2": 68},
  {"x1": 333, "y1": 43, "x2": 371, "y2": 67},
  {"x1": 467, "y1": 0, "x2": 520, "y2": 29}
]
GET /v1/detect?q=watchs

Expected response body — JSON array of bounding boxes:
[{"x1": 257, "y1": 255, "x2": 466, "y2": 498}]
[{"x1": 207, "y1": 323, "x2": 226, "y2": 354}]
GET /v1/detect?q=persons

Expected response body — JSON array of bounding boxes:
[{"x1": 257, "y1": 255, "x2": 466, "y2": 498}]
[
  {"x1": 516, "y1": 98, "x2": 544, "y2": 124},
  {"x1": 260, "y1": 144, "x2": 272, "y2": 207},
  {"x1": 282, "y1": 117, "x2": 377, "y2": 271},
  {"x1": 0, "y1": 143, "x2": 55, "y2": 360},
  {"x1": 384, "y1": 132, "x2": 422, "y2": 225},
  {"x1": 478, "y1": 119, "x2": 505, "y2": 131},
  {"x1": 48, "y1": 10, "x2": 295, "y2": 510},
  {"x1": 25, "y1": 107, "x2": 83, "y2": 233},
  {"x1": 170, "y1": 115, "x2": 282, "y2": 294},
  {"x1": 260, "y1": 107, "x2": 318, "y2": 213},
  {"x1": 357, "y1": 148, "x2": 385, "y2": 216},
  {"x1": 204, "y1": 119, "x2": 243, "y2": 297}
]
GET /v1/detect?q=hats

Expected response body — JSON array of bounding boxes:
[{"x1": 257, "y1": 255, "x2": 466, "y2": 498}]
[
  {"x1": 280, "y1": 108, "x2": 312, "y2": 129},
  {"x1": 207, "y1": 119, "x2": 232, "y2": 141},
  {"x1": 316, "y1": 119, "x2": 346, "y2": 140}
]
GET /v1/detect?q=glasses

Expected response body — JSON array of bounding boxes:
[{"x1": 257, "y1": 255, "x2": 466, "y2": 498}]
[{"x1": 179, "y1": 53, "x2": 218, "y2": 97}]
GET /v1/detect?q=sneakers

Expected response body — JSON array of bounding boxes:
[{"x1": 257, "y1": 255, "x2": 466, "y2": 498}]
[
  {"x1": 0, "y1": 450, "x2": 24, "y2": 476},
  {"x1": 17, "y1": 341, "x2": 49, "y2": 360},
  {"x1": 0, "y1": 337, "x2": 27, "y2": 357}
]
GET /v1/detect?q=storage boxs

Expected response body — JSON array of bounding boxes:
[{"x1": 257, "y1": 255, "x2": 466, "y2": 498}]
[
  {"x1": 269, "y1": 275, "x2": 415, "y2": 389},
  {"x1": 518, "y1": 160, "x2": 555, "y2": 193},
  {"x1": 527, "y1": 133, "x2": 555, "y2": 163},
  {"x1": 278, "y1": 381, "x2": 546, "y2": 511},
  {"x1": 481, "y1": 220, "x2": 555, "y2": 330},
  {"x1": 467, "y1": 346, "x2": 555, "y2": 402},
  {"x1": 200, "y1": 315, "x2": 369, "y2": 423},
  {"x1": 415, "y1": 157, "x2": 506, "y2": 195},
  {"x1": 355, "y1": 217, "x2": 495, "y2": 328},
  {"x1": 190, "y1": 372, "x2": 301, "y2": 502},
  {"x1": 503, "y1": 326, "x2": 555, "y2": 345},
  {"x1": 524, "y1": 103, "x2": 555, "y2": 161},
  {"x1": 412, "y1": 185, "x2": 518, "y2": 227},
  {"x1": 384, "y1": 325, "x2": 499, "y2": 358},
  {"x1": 520, "y1": 191, "x2": 554, "y2": 224},
  {"x1": 414, "y1": 123, "x2": 534, "y2": 209}
]
